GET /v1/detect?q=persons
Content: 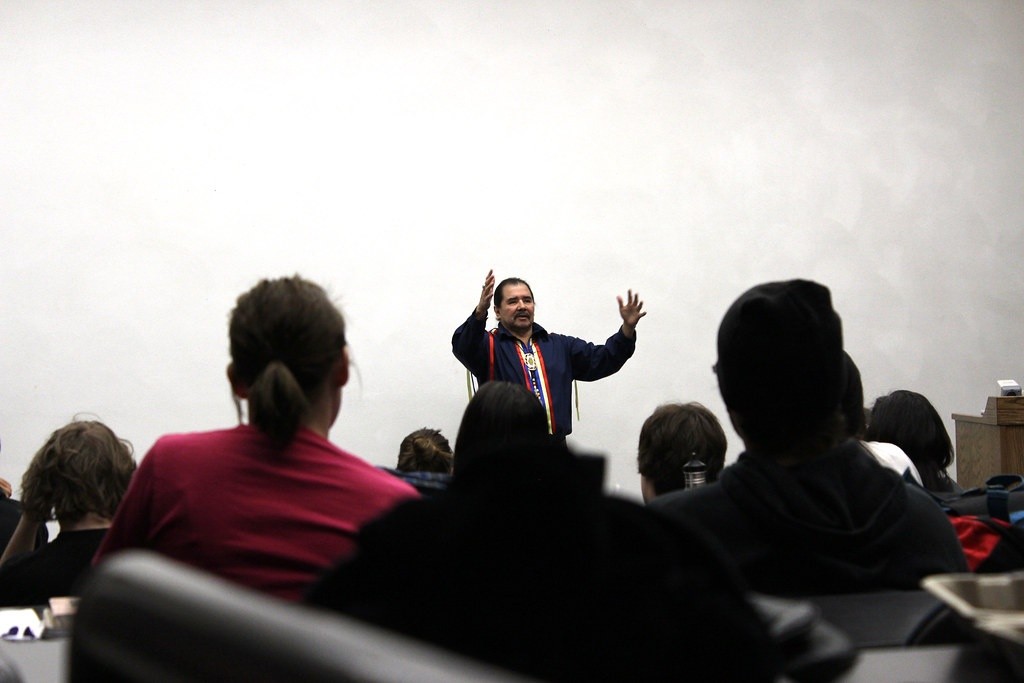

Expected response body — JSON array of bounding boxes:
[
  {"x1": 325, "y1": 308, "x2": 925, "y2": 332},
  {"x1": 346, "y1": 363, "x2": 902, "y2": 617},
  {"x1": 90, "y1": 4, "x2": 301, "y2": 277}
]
[
  {"x1": 0, "y1": 280, "x2": 1024, "y2": 683},
  {"x1": 451, "y1": 269, "x2": 647, "y2": 455}
]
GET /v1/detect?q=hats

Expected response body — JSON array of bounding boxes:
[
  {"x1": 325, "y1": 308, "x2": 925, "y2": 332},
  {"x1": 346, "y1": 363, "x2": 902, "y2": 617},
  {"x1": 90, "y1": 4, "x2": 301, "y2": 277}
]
[{"x1": 712, "y1": 278, "x2": 843, "y2": 431}]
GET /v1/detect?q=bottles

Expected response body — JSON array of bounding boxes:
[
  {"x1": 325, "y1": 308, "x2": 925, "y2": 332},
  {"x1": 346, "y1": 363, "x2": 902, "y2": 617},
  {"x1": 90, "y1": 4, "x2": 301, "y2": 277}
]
[{"x1": 681, "y1": 452, "x2": 709, "y2": 491}]
